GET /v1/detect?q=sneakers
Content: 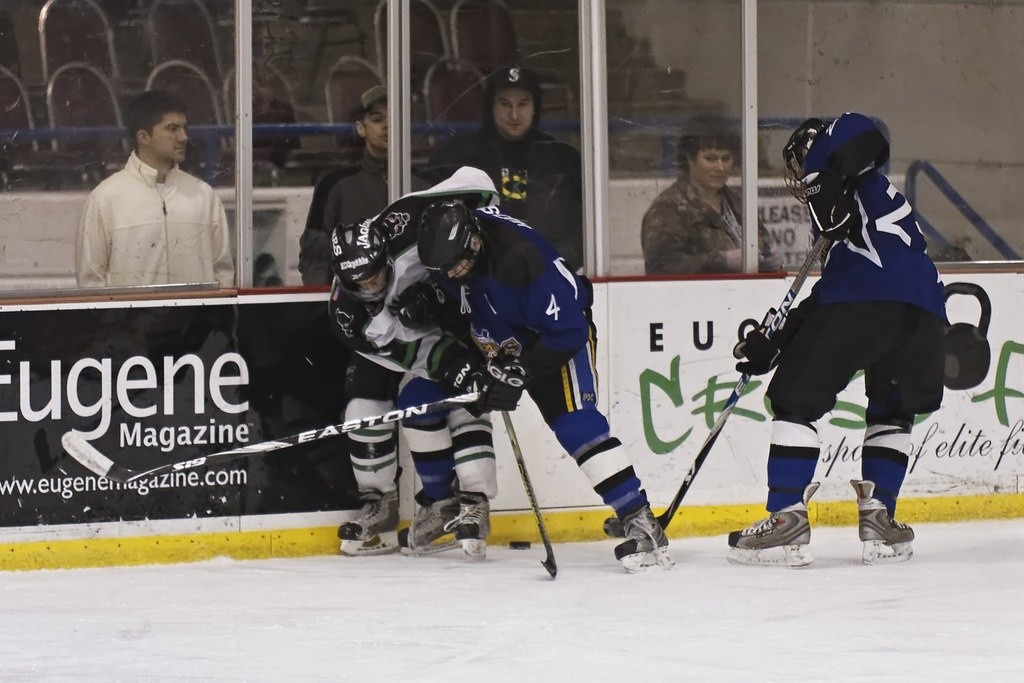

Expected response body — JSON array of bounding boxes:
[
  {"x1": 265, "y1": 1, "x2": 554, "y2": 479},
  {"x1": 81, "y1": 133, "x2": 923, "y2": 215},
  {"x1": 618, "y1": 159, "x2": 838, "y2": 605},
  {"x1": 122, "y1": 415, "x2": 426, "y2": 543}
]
[
  {"x1": 727, "y1": 482, "x2": 821, "y2": 566},
  {"x1": 850, "y1": 480, "x2": 915, "y2": 562},
  {"x1": 614, "y1": 489, "x2": 675, "y2": 573},
  {"x1": 337, "y1": 490, "x2": 399, "y2": 554},
  {"x1": 444, "y1": 490, "x2": 491, "y2": 556},
  {"x1": 401, "y1": 489, "x2": 462, "y2": 554}
]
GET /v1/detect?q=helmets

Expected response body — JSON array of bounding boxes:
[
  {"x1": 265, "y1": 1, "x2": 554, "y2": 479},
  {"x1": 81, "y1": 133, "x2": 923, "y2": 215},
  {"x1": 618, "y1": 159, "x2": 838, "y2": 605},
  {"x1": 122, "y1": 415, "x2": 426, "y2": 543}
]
[
  {"x1": 783, "y1": 118, "x2": 830, "y2": 171},
  {"x1": 417, "y1": 201, "x2": 480, "y2": 279},
  {"x1": 331, "y1": 217, "x2": 395, "y2": 303}
]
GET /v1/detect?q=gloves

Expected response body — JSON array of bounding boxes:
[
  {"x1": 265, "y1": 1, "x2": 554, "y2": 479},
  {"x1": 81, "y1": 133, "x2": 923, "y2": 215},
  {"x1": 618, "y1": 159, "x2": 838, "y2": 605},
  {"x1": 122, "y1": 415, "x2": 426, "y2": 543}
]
[
  {"x1": 734, "y1": 308, "x2": 794, "y2": 375},
  {"x1": 387, "y1": 281, "x2": 438, "y2": 328},
  {"x1": 442, "y1": 362, "x2": 491, "y2": 418},
  {"x1": 800, "y1": 172, "x2": 852, "y2": 241},
  {"x1": 481, "y1": 355, "x2": 526, "y2": 410}
]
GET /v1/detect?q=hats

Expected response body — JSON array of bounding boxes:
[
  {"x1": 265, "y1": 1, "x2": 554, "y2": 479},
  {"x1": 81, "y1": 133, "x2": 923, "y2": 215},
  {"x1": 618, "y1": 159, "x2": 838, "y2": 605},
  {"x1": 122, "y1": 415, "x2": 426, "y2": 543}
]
[
  {"x1": 486, "y1": 68, "x2": 540, "y2": 93},
  {"x1": 361, "y1": 84, "x2": 388, "y2": 109}
]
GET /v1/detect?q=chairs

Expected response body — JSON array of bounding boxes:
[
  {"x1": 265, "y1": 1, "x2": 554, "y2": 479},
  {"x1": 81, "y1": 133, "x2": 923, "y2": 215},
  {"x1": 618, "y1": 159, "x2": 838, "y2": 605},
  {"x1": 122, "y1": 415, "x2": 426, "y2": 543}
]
[{"x1": 0, "y1": 0, "x2": 765, "y2": 190}]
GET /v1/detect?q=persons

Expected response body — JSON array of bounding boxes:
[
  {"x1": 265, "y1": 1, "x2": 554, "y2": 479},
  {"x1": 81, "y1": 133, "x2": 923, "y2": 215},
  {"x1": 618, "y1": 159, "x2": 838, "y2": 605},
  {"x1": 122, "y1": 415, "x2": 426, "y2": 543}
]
[
  {"x1": 429, "y1": 66, "x2": 583, "y2": 273},
  {"x1": 640, "y1": 116, "x2": 784, "y2": 273},
  {"x1": 388, "y1": 203, "x2": 676, "y2": 575},
  {"x1": 330, "y1": 166, "x2": 500, "y2": 563},
  {"x1": 75, "y1": 90, "x2": 234, "y2": 287},
  {"x1": 299, "y1": 85, "x2": 389, "y2": 286},
  {"x1": 727, "y1": 112, "x2": 948, "y2": 567}
]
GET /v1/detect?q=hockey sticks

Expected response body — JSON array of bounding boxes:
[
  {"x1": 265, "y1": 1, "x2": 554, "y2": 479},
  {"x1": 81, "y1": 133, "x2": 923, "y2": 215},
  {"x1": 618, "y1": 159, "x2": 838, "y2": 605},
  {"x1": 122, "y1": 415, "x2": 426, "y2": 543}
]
[
  {"x1": 502, "y1": 410, "x2": 559, "y2": 581},
  {"x1": 614, "y1": 175, "x2": 860, "y2": 561},
  {"x1": 60, "y1": 390, "x2": 481, "y2": 485}
]
